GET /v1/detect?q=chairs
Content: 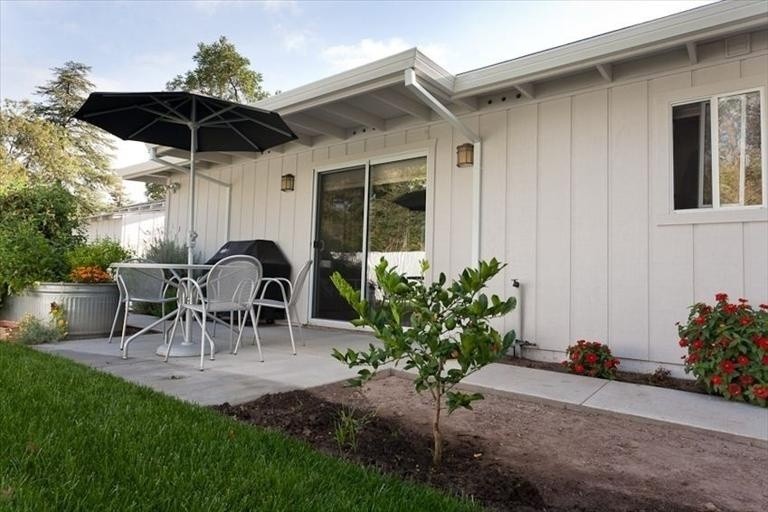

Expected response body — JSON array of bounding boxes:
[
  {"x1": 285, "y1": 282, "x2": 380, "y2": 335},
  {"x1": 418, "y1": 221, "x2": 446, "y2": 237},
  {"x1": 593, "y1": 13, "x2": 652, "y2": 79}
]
[{"x1": 108, "y1": 254, "x2": 312, "y2": 372}]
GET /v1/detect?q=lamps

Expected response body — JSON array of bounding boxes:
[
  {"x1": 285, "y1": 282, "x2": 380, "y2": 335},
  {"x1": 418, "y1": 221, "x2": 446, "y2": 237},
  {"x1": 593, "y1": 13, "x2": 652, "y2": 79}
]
[
  {"x1": 281, "y1": 174, "x2": 294, "y2": 193},
  {"x1": 456, "y1": 143, "x2": 473, "y2": 168}
]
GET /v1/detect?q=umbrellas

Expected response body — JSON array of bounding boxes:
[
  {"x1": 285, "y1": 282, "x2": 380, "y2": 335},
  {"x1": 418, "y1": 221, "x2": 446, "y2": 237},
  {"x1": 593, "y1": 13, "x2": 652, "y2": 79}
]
[{"x1": 71, "y1": 92, "x2": 300, "y2": 345}]
